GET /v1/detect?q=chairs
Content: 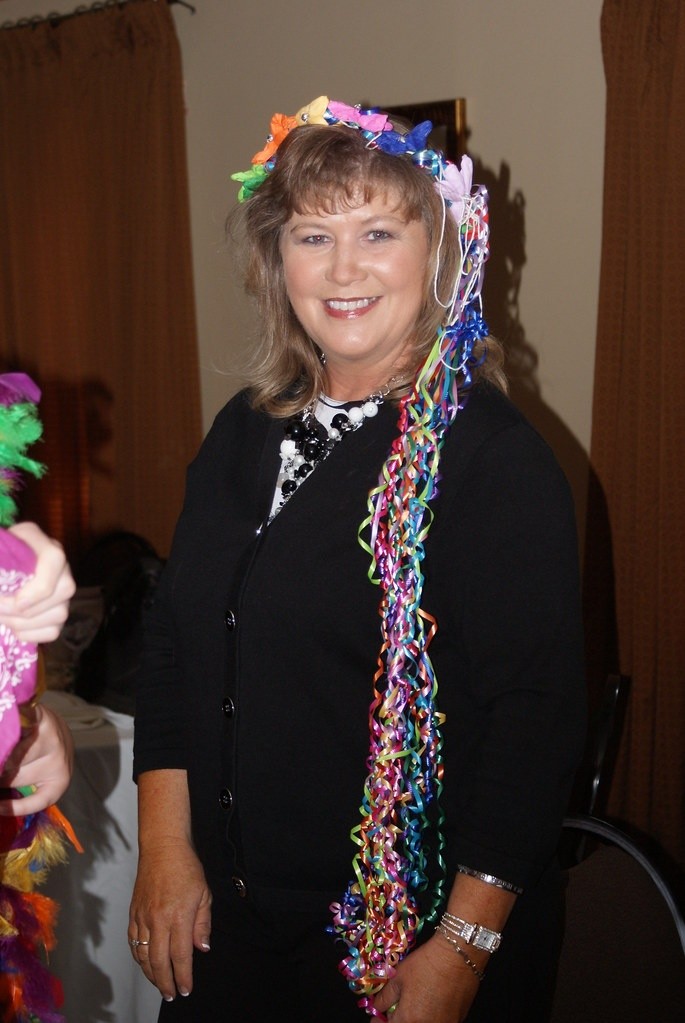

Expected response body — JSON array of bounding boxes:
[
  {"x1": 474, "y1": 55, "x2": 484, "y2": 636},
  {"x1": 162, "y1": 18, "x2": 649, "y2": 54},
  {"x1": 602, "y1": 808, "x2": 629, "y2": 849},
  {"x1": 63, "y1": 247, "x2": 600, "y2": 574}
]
[{"x1": 549, "y1": 813, "x2": 685, "y2": 1023}]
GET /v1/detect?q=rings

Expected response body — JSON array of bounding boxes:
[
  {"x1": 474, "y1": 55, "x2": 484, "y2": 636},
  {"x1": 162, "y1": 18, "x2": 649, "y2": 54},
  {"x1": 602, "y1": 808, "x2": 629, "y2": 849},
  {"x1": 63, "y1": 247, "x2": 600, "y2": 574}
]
[{"x1": 131, "y1": 940, "x2": 148, "y2": 947}]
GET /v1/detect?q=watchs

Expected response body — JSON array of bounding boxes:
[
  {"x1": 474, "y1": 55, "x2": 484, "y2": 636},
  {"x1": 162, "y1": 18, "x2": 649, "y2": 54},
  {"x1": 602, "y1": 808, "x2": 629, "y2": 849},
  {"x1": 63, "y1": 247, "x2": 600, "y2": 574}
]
[{"x1": 441, "y1": 913, "x2": 502, "y2": 952}]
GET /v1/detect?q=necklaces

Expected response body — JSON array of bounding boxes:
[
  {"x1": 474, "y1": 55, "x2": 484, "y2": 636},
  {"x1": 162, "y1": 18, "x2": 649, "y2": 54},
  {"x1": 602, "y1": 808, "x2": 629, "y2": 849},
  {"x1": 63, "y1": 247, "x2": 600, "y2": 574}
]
[{"x1": 268, "y1": 352, "x2": 409, "y2": 522}]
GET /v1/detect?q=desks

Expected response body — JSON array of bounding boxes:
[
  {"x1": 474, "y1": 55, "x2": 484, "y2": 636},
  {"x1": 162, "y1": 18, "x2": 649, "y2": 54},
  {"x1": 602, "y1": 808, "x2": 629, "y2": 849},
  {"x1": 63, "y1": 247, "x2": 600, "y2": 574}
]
[{"x1": 45, "y1": 691, "x2": 163, "y2": 1023}]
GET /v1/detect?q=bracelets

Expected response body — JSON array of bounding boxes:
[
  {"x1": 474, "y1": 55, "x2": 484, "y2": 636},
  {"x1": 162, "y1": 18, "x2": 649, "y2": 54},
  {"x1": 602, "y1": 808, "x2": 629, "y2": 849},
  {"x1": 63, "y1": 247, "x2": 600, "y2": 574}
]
[
  {"x1": 436, "y1": 927, "x2": 485, "y2": 981},
  {"x1": 457, "y1": 865, "x2": 522, "y2": 895}
]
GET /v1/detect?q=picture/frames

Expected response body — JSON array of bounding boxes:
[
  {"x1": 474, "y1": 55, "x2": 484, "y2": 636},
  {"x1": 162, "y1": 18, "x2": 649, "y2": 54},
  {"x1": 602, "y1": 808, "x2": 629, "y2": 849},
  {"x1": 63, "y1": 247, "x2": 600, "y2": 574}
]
[{"x1": 379, "y1": 97, "x2": 465, "y2": 168}]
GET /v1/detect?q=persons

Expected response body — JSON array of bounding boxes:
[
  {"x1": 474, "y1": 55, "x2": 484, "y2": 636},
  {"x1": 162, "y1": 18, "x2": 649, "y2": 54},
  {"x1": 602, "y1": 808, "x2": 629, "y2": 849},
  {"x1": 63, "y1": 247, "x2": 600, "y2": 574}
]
[
  {"x1": 0, "y1": 519, "x2": 75, "y2": 818},
  {"x1": 125, "y1": 102, "x2": 590, "y2": 1023}
]
